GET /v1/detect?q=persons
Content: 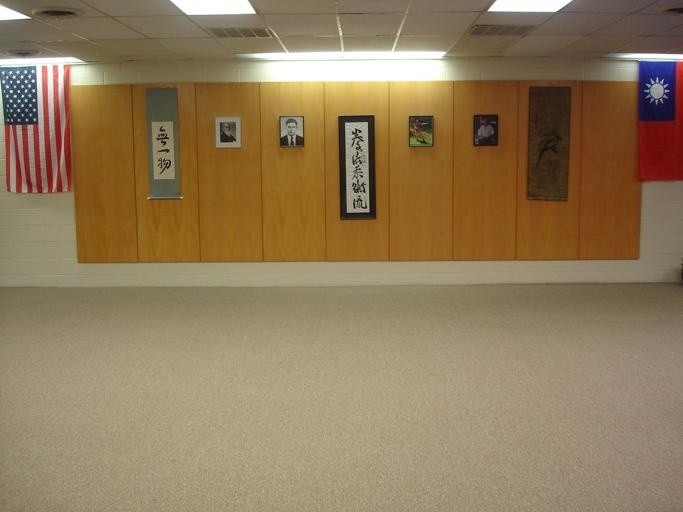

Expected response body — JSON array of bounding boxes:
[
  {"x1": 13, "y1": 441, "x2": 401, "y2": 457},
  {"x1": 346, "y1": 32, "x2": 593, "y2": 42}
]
[
  {"x1": 412, "y1": 119, "x2": 426, "y2": 144},
  {"x1": 476, "y1": 116, "x2": 494, "y2": 142},
  {"x1": 219, "y1": 122, "x2": 235, "y2": 143}
]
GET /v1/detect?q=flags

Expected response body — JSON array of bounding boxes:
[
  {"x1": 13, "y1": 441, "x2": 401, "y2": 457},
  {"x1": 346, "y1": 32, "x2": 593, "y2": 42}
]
[
  {"x1": 637, "y1": 57, "x2": 683, "y2": 181},
  {"x1": 0, "y1": 63, "x2": 72, "y2": 196}
]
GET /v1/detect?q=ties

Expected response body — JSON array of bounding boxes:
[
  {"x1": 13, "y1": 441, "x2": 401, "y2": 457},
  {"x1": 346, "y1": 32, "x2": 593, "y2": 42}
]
[{"x1": 290, "y1": 137, "x2": 293, "y2": 145}]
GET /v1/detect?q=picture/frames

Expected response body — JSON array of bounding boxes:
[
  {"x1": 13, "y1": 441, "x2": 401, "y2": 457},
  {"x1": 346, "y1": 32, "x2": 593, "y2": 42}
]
[
  {"x1": 214, "y1": 116, "x2": 242, "y2": 149},
  {"x1": 338, "y1": 115, "x2": 376, "y2": 220},
  {"x1": 409, "y1": 116, "x2": 433, "y2": 147},
  {"x1": 473, "y1": 115, "x2": 498, "y2": 146}
]
